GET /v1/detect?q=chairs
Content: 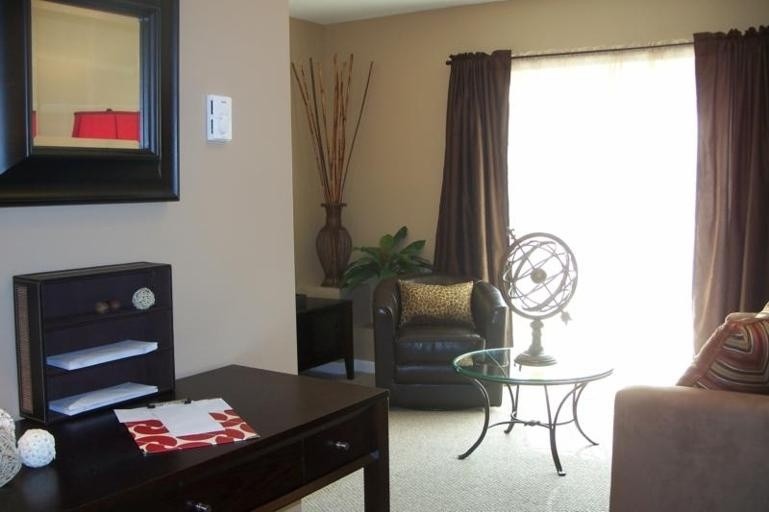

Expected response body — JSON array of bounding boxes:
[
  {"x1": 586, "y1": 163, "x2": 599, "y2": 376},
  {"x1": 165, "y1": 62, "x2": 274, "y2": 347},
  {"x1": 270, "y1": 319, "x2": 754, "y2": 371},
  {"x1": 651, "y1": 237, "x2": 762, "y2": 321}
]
[
  {"x1": 607, "y1": 386, "x2": 769, "y2": 510},
  {"x1": 371, "y1": 275, "x2": 507, "y2": 409}
]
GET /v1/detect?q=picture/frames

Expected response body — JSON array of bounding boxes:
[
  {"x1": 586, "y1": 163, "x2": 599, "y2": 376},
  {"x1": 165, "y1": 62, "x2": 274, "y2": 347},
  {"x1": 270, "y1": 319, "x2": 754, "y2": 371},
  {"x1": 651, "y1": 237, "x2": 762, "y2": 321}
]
[{"x1": 1, "y1": 1, "x2": 182, "y2": 209}]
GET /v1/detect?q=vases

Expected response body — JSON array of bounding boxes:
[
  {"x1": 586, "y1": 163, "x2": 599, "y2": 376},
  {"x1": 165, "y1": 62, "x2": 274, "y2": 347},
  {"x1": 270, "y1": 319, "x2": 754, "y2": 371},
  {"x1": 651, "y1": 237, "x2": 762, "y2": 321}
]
[{"x1": 316, "y1": 203, "x2": 354, "y2": 289}]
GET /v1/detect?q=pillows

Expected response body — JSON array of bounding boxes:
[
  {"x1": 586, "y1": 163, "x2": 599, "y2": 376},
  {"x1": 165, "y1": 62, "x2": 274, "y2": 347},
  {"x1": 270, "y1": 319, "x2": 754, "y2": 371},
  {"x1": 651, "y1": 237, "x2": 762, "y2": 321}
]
[
  {"x1": 676, "y1": 300, "x2": 769, "y2": 394},
  {"x1": 396, "y1": 278, "x2": 476, "y2": 330}
]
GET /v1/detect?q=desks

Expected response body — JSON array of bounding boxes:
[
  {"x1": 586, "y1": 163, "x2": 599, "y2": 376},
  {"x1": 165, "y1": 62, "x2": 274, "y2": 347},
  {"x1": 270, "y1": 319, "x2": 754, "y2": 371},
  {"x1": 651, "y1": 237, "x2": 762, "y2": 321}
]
[
  {"x1": 450, "y1": 347, "x2": 613, "y2": 477},
  {"x1": 296, "y1": 294, "x2": 356, "y2": 379},
  {"x1": 1, "y1": 362, "x2": 392, "y2": 512}
]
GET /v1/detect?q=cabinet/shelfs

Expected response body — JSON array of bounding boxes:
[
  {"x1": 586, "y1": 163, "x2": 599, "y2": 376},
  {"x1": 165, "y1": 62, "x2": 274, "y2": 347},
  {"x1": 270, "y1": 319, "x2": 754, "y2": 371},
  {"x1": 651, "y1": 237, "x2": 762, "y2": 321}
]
[{"x1": 10, "y1": 259, "x2": 178, "y2": 425}]
